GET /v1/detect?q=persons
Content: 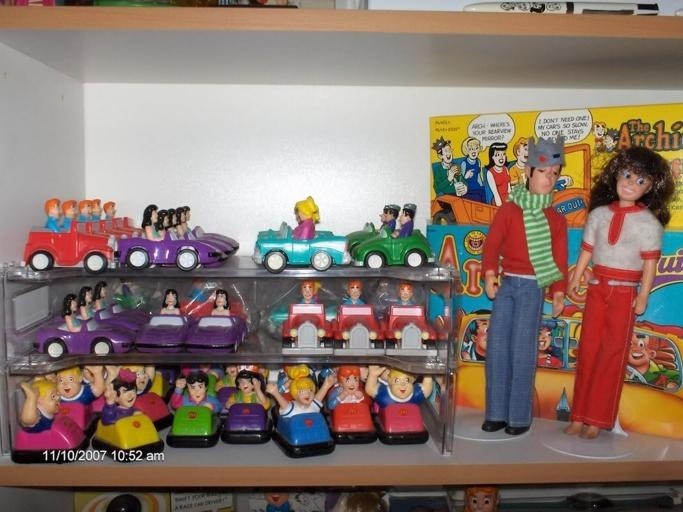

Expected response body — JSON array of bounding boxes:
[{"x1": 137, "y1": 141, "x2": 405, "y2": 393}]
[
  {"x1": 224, "y1": 370, "x2": 270, "y2": 410},
  {"x1": 364, "y1": 364, "x2": 433, "y2": 407},
  {"x1": 101, "y1": 368, "x2": 137, "y2": 426},
  {"x1": 183, "y1": 364, "x2": 220, "y2": 398},
  {"x1": 266, "y1": 374, "x2": 337, "y2": 419},
  {"x1": 278, "y1": 364, "x2": 309, "y2": 394},
  {"x1": 105, "y1": 363, "x2": 156, "y2": 396},
  {"x1": 216, "y1": 364, "x2": 240, "y2": 391},
  {"x1": 320, "y1": 365, "x2": 340, "y2": 380},
  {"x1": 45, "y1": 364, "x2": 105, "y2": 406},
  {"x1": 171, "y1": 370, "x2": 222, "y2": 415},
  {"x1": 17, "y1": 376, "x2": 61, "y2": 434},
  {"x1": 327, "y1": 365, "x2": 365, "y2": 409}
]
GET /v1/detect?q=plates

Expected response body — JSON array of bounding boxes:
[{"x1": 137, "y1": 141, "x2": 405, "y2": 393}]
[
  {"x1": 0, "y1": 1, "x2": 683, "y2": 511},
  {"x1": 0, "y1": 256, "x2": 459, "y2": 456}
]
